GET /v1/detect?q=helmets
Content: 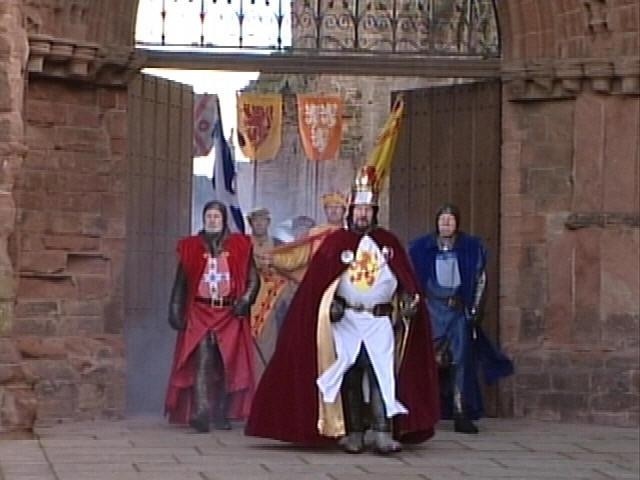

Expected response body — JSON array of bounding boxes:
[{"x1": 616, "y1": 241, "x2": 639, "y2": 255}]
[
  {"x1": 347, "y1": 165, "x2": 382, "y2": 208},
  {"x1": 245, "y1": 207, "x2": 271, "y2": 222}
]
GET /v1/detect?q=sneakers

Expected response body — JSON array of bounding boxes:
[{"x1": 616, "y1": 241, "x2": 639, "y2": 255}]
[
  {"x1": 212, "y1": 410, "x2": 231, "y2": 430},
  {"x1": 452, "y1": 417, "x2": 480, "y2": 435},
  {"x1": 366, "y1": 429, "x2": 403, "y2": 454},
  {"x1": 340, "y1": 432, "x2": 364, "y2": 454},
  {"x1": 187, "y1": 411, "x2": 209, "y2": 431}
]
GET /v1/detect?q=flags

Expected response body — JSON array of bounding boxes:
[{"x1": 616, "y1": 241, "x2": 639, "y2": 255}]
[
  {"x1": 296, "y1": 93, "x2": 345, "y2": 161},
  {"x1": 363, "y1": 91, "x2": 406, "y2": 197},
  {"x1": 208, "y1": 121, "x2": 249, "y2": 234},
  {"x1": 192, "y1": 93, "x2": 223, "y2": 159},
  {"x1": 234, "y1": 89, "x2": 284, "y2": 166}
]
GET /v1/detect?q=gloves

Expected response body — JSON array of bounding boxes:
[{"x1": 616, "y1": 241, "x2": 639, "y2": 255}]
[
  {"x1": 328, "y1": 301, "x2": 343, "y2": 323},
  {"x1": 168, "y1": 309, "x2": 186, "y2": 330},
  {"x1": 234, "y1": 299, "x2": 249, "y2": 316}
]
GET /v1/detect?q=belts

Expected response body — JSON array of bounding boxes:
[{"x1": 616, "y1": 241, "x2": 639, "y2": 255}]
[{"x1": 194, "y1": 294, "x2": 230, "y2": 309}]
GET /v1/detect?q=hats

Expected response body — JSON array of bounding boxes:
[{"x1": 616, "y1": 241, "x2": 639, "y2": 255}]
[
  {"x1": 204, "y1": 201, "x2": 225, "y2": 213},
  {"x1": 319, "y1": 190, "x2": 348, "y2": 211},
  {"x1": 435, "y1": 203, "x2": 461, "y2": 218}
]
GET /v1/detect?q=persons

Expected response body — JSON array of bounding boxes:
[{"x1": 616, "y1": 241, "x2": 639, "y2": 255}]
[
  {"x1": 312, "y1": 204, "x2": 422, "y2": 454},
  {"x1": 162, "y1": 199, "x2": 261, "y2": 434},
  {"x1": 406, "y1": 203, "x2": 515, "y2": 434},
  {"x1": 283, "y1": 215, "x2": 316, "y2": 245},
  {"x1": 258, "y1": 190, "x2": 347, "y2": 286},
  {"x1": 238, "y1": 206, "x2": 291, "y2": 336}
]
